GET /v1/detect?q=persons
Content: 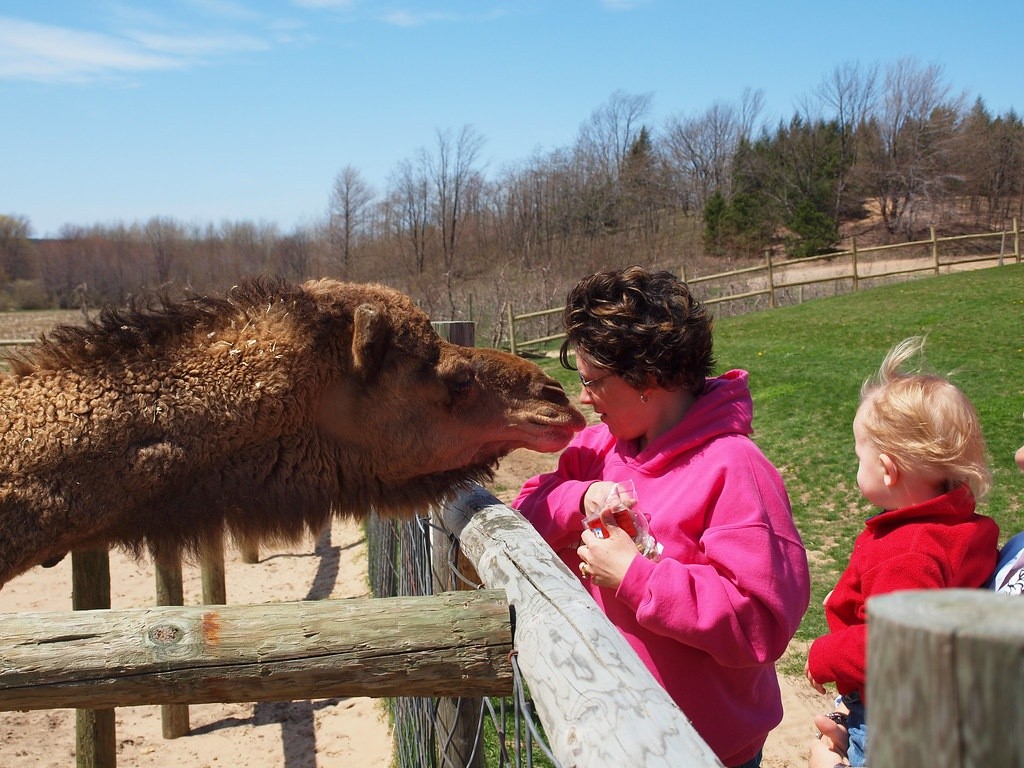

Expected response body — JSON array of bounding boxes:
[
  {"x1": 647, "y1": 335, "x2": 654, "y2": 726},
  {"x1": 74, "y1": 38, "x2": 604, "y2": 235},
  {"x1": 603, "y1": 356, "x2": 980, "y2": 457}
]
[
  {"x1": 799, "y1": 372, "x2": 1023, "y2": 767},
  {"x1": 509, "y1": 265, "x2": 812, "y2": 767}
]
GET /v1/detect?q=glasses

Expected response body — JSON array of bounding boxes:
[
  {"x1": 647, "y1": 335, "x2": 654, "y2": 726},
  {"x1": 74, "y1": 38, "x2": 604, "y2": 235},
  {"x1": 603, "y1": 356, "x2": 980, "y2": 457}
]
[{"x1": 580, "y1": 370, "x2": 620, "y2": 392}]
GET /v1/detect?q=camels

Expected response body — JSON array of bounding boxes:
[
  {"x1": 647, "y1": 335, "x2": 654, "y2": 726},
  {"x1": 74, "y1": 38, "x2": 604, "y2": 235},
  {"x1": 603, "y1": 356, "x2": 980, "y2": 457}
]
[{"x1": 0, "y1": 278, "x2": 586, "y2": 594}]
